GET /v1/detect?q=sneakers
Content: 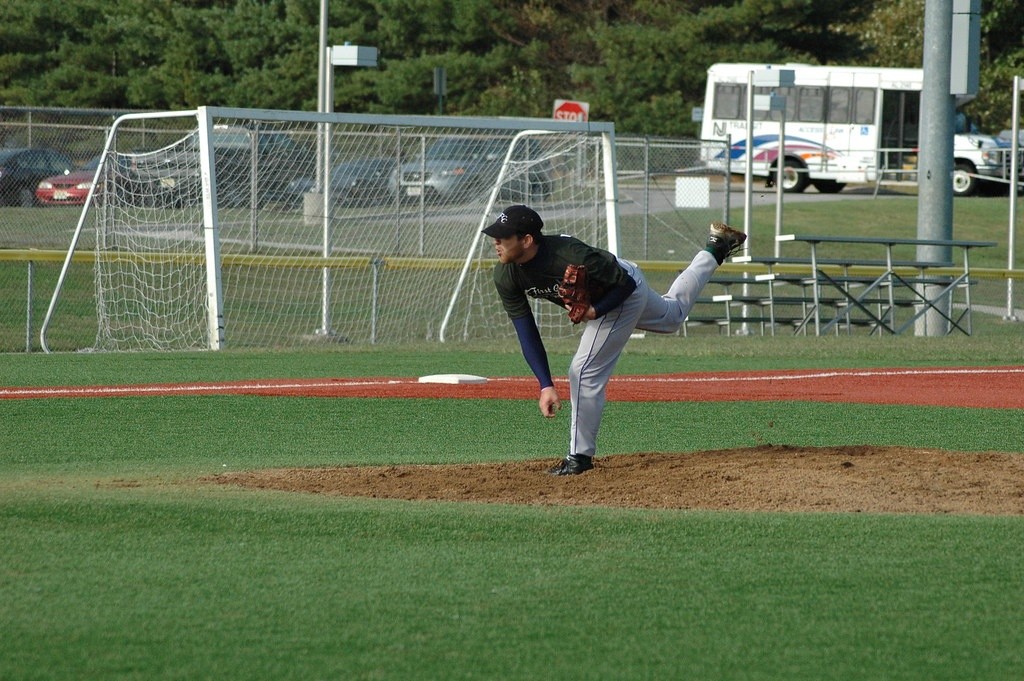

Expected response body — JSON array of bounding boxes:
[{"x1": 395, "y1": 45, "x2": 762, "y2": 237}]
[
  {"x1": 706, "y1": 222, "x2": 747, "y2": 256},
  {"x1": 546, "y1": 454, "x2": 593, "y2": 476}
]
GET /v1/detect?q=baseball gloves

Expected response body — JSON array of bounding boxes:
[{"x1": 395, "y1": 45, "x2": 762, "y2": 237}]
[{"x1": 556, "y1": 264, "x2": 590, "y2": 325}]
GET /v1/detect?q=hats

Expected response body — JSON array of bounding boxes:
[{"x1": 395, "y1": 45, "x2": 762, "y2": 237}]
[{"x1": 481, "y1": 204, "x2": 543, "y2": 238}]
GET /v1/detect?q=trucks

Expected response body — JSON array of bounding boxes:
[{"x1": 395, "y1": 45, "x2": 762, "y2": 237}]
[{"x1": 701, "y1": 60, "x2": 1002, "y2": 198}]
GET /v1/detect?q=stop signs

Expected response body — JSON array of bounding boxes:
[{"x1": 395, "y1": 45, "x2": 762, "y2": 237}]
[{"x1": 552, "y1": 99, "x2": 589, "y2": 125}]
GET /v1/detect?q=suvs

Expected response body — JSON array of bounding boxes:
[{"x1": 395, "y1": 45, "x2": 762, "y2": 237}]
[{"x1": 130, "y1": 126, "x2": 309, "y2": 209}]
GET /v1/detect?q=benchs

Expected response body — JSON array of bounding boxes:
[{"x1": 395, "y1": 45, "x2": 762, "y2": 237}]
[{"x1": 656, "y1": 232, "x2": 999, "y2": 338}]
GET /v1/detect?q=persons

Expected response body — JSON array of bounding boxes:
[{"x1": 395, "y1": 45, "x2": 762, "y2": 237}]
[{"x1": 482, "y1": 205, "x2": 747, "y2": 476}]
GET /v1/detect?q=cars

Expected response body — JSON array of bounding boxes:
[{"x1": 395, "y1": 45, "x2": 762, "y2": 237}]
[
  {"x1": 388, "y1": 134, "x2": 556, "y2": 205},
  {"x1": 288, "y1": 156, "x2": 406, "y2": 210},
  {"x1": 1, "y1": 147, "x2": 80, "y2": 209},
  {"x1": 35, "y1": 156, "x2": 135, "y2": 207}
]
